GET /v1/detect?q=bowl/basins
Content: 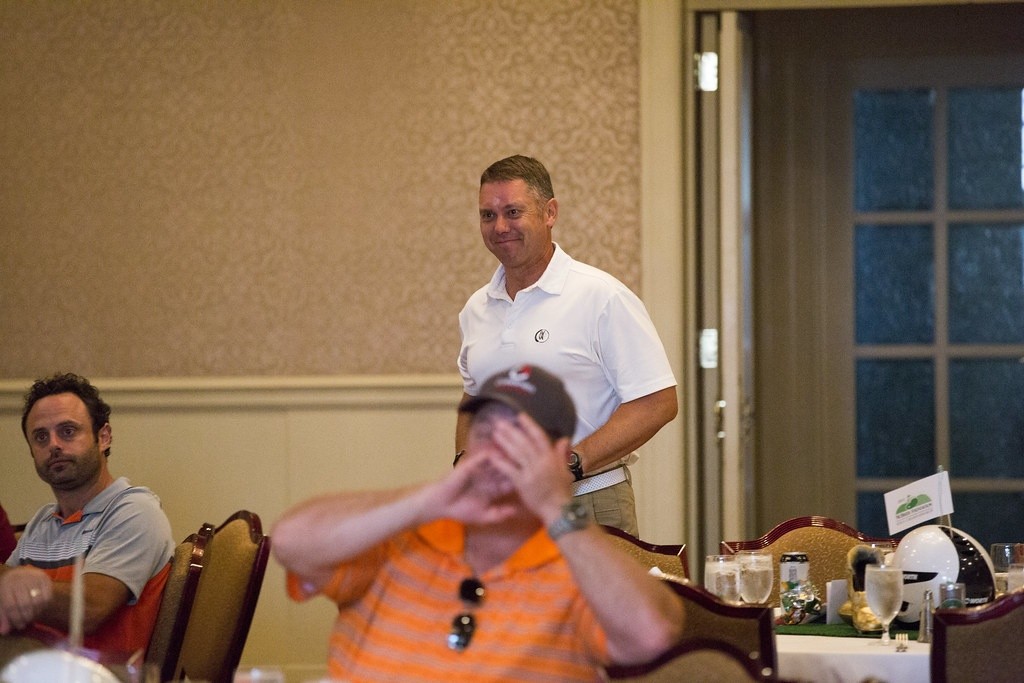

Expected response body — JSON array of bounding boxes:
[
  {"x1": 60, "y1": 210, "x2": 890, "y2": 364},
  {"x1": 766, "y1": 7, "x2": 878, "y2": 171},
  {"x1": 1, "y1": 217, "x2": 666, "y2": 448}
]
[{"x1": 838, "y1": 613, "x2": 855, "y2": 627}]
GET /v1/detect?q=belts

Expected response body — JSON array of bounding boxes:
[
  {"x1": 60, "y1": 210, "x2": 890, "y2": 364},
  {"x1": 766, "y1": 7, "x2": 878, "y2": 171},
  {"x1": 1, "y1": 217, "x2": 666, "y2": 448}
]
[{"x1": 570, "y1": 465, "x2": 627, "y2": 501}]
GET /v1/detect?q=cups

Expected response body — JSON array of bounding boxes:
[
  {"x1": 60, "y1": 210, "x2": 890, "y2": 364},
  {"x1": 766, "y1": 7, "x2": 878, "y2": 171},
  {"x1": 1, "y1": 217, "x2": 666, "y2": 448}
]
[
  {"x1": 849, "y1": 571, "x2": 884, "y2": 633},
  {"x1": 737, "y1": 549, "x2": 772, "y2": 605},
  {"x1": 939, "y1": 584, "x2": 965, "y2": 608},
  {"x1": 705, "y1": 555, "x2": 740, "y2": 605},
  {"x1": 990, "y1": 543, "x2": 1018, "y2": 596},
  {"x1": 1008, "y1": 563, "x2": 1024, "y2": 589}
]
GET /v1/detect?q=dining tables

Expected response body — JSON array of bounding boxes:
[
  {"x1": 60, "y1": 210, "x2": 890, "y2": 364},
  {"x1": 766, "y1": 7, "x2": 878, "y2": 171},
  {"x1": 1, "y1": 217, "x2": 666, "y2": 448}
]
[{"x1": 746, "y1": 605, "x2": 938, "y2": 683}]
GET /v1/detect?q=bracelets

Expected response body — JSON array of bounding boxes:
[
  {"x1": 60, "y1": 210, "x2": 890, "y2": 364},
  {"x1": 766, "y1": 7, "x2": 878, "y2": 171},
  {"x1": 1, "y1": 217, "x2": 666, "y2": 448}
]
[{"x1": 453, "y1": 450, "x2": 466, "y2": 470}]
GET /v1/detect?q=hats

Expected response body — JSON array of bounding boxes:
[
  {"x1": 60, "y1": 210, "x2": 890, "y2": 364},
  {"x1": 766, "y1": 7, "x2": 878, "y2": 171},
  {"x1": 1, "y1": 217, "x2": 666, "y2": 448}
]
[{"x1": 458, "y1": 366, "x2": 579, "y2": 447}]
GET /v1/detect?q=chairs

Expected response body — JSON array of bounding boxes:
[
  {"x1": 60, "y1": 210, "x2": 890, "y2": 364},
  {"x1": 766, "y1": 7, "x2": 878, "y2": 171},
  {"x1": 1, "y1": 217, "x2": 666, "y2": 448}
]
[
  {"x1": 598, "y1": 515, "x2": 1024, "y2": 683},
  {"x1": 0, "y1": 509, "x2": 274, "y2": 683}
]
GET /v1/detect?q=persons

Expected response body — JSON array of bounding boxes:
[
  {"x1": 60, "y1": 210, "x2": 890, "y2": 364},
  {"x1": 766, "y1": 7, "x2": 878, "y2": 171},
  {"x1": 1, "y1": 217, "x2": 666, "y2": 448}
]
[
  {"x1": 453, "y1": 155, "x2": 678, "y2": 540},
  {"x1": 271, "y1": 365, "x2": 685, "y2": 683},
  {"x1": 0, "y1": 372, "x2": 174, "y2": 683}
]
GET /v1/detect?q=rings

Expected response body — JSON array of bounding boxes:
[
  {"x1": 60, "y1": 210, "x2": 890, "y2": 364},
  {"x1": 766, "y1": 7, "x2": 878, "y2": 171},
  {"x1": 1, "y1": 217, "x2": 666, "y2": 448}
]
[{"x1": 30, "y1": 589, "x2": 41, "y2": 597}]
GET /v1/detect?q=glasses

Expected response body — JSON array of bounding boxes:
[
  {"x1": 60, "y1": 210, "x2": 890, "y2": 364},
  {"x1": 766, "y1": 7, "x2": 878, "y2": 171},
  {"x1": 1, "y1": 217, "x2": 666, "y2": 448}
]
[{"x1": 439, "y1": 571, "x2": 485, "y2": 652}]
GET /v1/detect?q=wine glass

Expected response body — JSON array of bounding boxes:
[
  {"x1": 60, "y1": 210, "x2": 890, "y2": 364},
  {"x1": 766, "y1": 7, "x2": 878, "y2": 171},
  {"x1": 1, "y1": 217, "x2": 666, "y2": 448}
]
[{"x1": 865, "y1": 563, "x2": 904, "y2": 645}]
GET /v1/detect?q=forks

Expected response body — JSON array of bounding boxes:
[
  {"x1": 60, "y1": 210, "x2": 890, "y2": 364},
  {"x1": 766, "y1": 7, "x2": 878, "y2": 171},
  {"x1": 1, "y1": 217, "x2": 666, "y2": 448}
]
[{"x1": 896, "y1": 634, "x2": 909, "y2": 650}]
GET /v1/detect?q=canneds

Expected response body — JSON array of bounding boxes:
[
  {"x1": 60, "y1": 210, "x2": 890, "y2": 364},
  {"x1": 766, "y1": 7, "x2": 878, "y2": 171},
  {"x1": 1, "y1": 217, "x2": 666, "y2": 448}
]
[{"x1": 778, "y1": 551, "x2": 810, "y2": 619}]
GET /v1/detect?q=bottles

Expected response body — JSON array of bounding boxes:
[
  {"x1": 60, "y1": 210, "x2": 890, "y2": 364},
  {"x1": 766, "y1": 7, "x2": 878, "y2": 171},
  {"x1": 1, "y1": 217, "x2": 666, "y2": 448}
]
[{"x1": 917, "y1": 589, "x2": 936, "y2": 642}]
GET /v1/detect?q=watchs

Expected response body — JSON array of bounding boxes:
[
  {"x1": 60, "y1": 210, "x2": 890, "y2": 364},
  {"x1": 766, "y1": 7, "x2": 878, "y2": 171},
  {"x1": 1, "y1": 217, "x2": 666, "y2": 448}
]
[
  {"x1": 548, "y1": 504, "x2": 591, "y2": 542},
  {"x1": 567, "y1": 451, "x2": 583, "y2": 482}
]
